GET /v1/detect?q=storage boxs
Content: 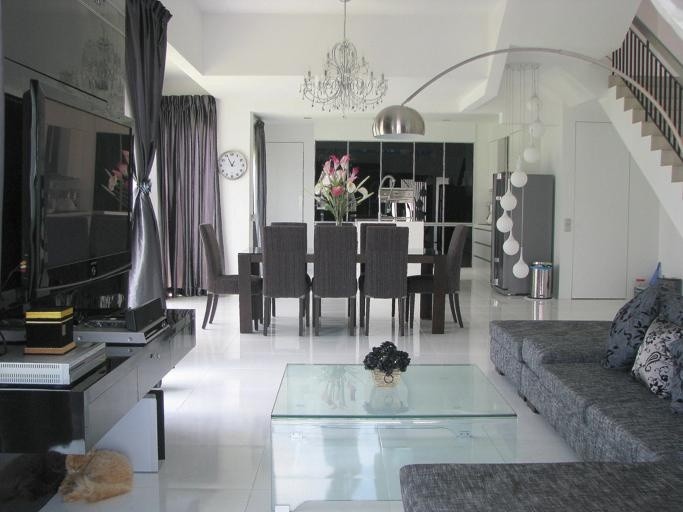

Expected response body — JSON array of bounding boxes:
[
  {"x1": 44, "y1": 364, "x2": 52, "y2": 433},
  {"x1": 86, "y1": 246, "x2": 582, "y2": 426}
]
[{"x1": 22, "y1": 305, "x2": 77, "y2": 355}]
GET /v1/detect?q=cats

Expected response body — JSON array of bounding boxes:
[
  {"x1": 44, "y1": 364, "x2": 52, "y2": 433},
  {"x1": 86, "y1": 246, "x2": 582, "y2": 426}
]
[{"x1": 56, "y1": 447, "x2": 133, "y2": 503}]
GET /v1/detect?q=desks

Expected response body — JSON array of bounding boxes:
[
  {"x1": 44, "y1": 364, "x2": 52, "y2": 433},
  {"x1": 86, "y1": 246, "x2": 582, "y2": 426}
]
[{"x1": 1, "y1": 309, "x2": 201, "y2": 463}]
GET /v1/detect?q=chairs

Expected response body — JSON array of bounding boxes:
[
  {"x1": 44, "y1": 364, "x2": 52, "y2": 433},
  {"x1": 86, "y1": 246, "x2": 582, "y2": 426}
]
[{"x1": 198, "y1": 221, "x2": 469, "y2": 340}]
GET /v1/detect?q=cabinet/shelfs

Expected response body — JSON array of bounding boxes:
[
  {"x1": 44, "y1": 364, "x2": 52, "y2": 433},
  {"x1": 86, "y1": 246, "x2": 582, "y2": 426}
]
[
  {"x1": 314, "y1": 140, "x2": 474, "y2": 269},
  {"x1": 471, "y1": 227, "x2": 491, "y2": 274}
]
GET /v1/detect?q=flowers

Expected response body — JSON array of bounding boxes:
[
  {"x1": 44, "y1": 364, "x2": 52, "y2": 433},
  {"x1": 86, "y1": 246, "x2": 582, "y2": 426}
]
[
  {"x1": 309, "y1": 153, "x2": 376, "y2": 215},
  {"x1": 361, "y1": 340, "x2": 411, "y2": 373},
  {"x1": 97, "y1": 144, "x2": 131, "y2": 211}
]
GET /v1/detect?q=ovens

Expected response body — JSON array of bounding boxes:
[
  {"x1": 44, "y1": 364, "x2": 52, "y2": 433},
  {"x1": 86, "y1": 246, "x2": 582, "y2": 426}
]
[{"x1": 378, "y1": 189, "x2": 414, "y2": 220}]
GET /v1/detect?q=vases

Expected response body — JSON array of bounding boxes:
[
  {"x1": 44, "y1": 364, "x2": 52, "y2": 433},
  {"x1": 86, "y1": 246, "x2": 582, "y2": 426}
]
[
  {"x1": 371, "y1": 368, "x2": 401, "y2": 387},
  {"x1": 332, "y1": 213, "x2": 344, "y2": 226}
]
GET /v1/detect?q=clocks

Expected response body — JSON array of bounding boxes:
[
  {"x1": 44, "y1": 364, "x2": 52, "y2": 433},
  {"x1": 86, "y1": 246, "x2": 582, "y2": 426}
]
[{"x1": 216, "y1": 149, "x2": 248, "y2": 181}]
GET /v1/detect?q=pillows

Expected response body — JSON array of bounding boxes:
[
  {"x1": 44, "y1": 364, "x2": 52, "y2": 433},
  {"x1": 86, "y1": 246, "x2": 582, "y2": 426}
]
[
  {"x1": 666, "y1": 339, "x2": 682, "y2": 415},
  {"x1": 629, "y1": 312, "x2": 681, "y2": 401},
  {"x1": 602, "y1": 284, "x2": 660, "y2": 371}
]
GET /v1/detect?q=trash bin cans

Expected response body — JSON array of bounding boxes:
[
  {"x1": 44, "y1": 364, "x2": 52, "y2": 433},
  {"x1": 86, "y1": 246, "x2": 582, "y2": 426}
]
[{"x1": 528, "y1": 261, "x2": 553, "y2": 299}]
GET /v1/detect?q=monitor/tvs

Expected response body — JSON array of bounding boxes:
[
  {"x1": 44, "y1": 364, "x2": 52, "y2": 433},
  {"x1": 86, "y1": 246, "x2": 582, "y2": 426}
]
[{"x1": 15, "y1": 78, "x2": 136, "y2": 294}]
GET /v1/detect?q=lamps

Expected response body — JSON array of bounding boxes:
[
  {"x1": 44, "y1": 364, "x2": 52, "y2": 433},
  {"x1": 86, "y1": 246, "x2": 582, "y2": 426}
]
[
  {"x1": 297, "y1": 2, "x2": 388, "y2": 120},
  {"x1": 493, "y1": 61, "x2": 543, "y2": 278},
  {"x1": 372, "y1": 46, "x2": 681, "y2": 160}
]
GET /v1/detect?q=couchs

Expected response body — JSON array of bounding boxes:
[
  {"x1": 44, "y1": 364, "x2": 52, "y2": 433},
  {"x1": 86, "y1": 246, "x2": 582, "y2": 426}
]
[{"x1": 399, "y1": 278, "x2": 682, "y2": 511}]
